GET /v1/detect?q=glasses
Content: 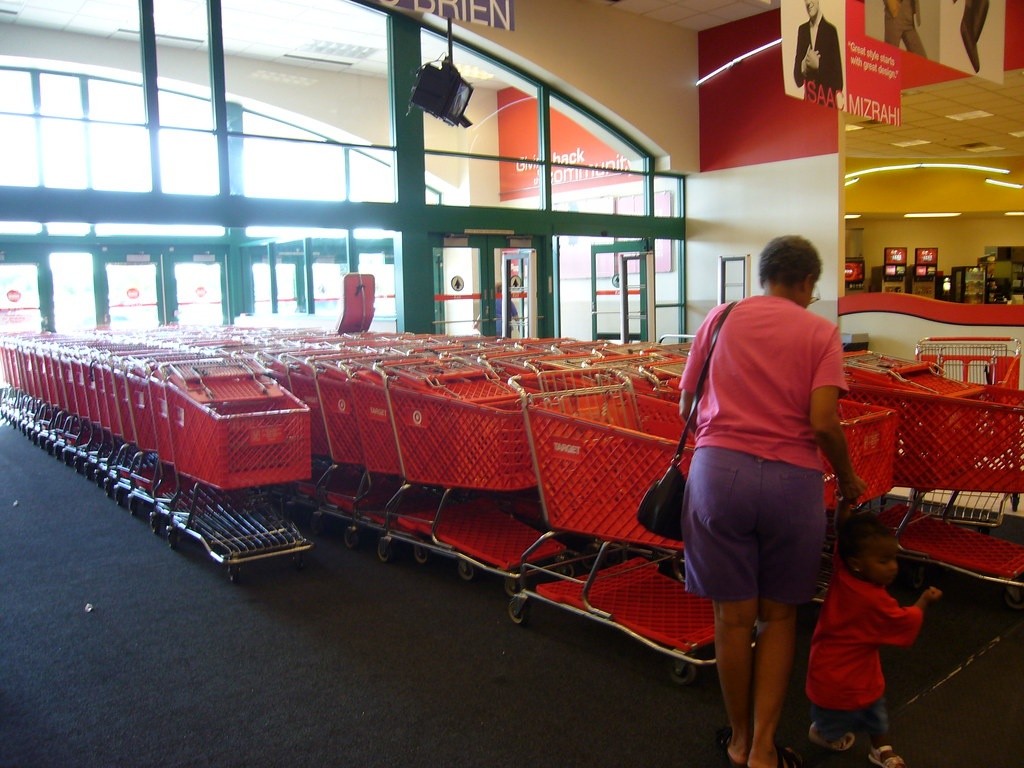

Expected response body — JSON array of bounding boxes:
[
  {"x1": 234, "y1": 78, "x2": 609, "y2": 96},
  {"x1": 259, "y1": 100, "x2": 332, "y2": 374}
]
[{"x1": 810, "y1": 283, "x2": 820, "y2": 304}]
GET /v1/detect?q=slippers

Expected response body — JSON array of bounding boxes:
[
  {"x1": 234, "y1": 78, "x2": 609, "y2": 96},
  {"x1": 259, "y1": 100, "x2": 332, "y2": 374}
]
[
  {"x1": 716, "y1": 723, "x2": 745, "y2": 768},
  {"x1": 775, "y1": 745, "x2": 804, "y2": 768}
]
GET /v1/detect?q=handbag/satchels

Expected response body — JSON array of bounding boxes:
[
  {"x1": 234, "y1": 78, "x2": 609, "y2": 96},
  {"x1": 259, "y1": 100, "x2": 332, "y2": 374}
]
[{"x1": 637, "y1": 462, "x2": 687, "y2": 542}]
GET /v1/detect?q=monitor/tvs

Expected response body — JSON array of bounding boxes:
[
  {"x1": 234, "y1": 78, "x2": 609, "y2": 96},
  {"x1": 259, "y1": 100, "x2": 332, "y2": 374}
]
[{"x1": 412, "y1": 65, "x2": 473, "y2": 125}]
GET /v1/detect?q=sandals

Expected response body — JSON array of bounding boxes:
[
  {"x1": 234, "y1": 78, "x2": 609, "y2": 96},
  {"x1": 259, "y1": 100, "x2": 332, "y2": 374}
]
[
  {"x1": 868, "y1": 745, "x2": 905, "y2": 768},
  {"x1": 808, "y1": 720, "x2": 855, "y2": 751}
]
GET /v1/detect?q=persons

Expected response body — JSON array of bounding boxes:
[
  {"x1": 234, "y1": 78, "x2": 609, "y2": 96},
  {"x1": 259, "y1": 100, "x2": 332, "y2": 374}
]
[
  {"x1": 678, "y1": 235, "x2": 858, "y2": 768},
  {"x1": 793, "y1": 0, "x2": 843, "y2": 110},
  {"x1": 473, "y1": 282, "x2": 522, "y2": 339},
  {"x1": 951, "y1": 0, "x2": 989, "y2": 82},
  {"x1": 804, "y1": 491, "x2": 942, "y2": 768},
  {"x1": 880, "y1": 0, "x2": 928, "y2": 59}
]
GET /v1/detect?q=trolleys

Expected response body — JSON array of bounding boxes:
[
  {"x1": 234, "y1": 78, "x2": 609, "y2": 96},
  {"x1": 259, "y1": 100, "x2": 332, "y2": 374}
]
[
  {"x1": 162, "y1": 315, "x2": 1024, "y2": 669},
  {"x1": 0, "y1": 321, "x2": 317, "y2": 587}
]
